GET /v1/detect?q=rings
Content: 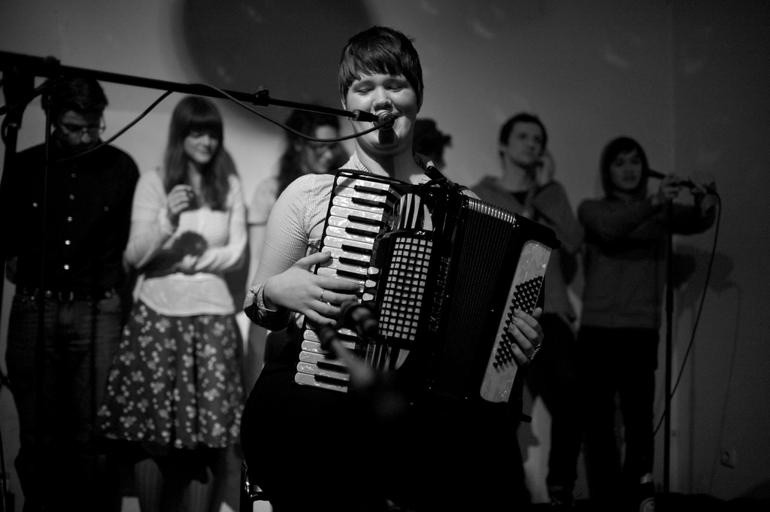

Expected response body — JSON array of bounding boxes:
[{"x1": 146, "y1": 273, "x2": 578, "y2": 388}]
[{"x1": 318, "y1": 288, "x2": 325, "y2": 301}]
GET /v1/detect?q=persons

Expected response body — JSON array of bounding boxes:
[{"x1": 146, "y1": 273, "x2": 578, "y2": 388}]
[
  {"x1": 1, "y1": 68, "x2": 141, "y2": 510},
  {"x1": 575, "y1": 134, "x2": 717, "y2": 509},
  {"x1": 464, "y1": 112, "x2": 589, "y2": 509},
  {"x1": 241, "y1": 24, "x2": 544, "y2": 510},
  {"x1": 81, "y1": 93, "x2": 249, "y2": 509},
  {"x1": 409, "y1": 121, "x2": 453, "y2": 169},
  {"x1": 246, "y1": 101, "x2": 343, "y2": 390}
]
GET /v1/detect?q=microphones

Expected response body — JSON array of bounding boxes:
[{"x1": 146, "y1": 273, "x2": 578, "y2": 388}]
[
  {"x1": 642, "y1": 168, "x2": 675, "y2": 187},
  {"x1": 375, "y1": 111, "x2": 396, "y2": 131}
]
